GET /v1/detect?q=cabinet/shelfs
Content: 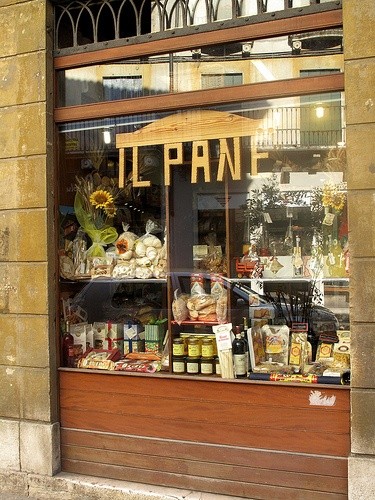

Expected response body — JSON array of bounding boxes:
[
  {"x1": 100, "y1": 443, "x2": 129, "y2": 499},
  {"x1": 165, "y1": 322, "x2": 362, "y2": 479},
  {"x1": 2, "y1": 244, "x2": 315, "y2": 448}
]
[{"x1": 165, "y1": 158, "x2": 231, "y2": 374}]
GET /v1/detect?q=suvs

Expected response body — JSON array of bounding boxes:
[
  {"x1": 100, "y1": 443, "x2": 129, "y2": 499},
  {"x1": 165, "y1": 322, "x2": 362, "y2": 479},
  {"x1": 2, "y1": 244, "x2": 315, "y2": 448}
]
[{"x1": 67, "y1": 273, "x2": 341, "y2": 357}]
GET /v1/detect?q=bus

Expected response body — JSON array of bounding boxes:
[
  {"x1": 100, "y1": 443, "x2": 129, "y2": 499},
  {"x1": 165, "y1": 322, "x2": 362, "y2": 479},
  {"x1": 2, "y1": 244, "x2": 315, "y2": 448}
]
[{"x1": 228, "y1": 278, "x2": 350, "y2": 330}]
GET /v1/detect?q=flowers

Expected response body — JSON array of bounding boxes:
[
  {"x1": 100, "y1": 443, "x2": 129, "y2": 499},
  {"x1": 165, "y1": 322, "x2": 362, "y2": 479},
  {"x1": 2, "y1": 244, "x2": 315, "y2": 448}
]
[
  {"x1": 322, "y1": 186, "x2": 347, "y2": 215},
  {"x1": 76, "y1": 175, "x2": 117, "y2": 229}
]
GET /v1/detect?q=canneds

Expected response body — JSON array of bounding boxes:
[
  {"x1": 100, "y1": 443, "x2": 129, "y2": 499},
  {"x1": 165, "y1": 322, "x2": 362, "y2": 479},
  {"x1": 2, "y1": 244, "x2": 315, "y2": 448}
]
[{"x1": 172, "y1": 337, "x2": 221, "y2": 377}]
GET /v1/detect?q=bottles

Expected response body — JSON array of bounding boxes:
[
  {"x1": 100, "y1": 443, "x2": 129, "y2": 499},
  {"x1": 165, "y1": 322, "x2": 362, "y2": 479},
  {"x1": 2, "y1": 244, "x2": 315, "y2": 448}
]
[
  {"x1": 232, "y1": 316, "x2": 252, "y2": 379},
  {"x1": 61, "y1": 320, "x2": 74, "y2": 368},
  {"x1": 172, "y1": 335, "x2": 221, "y2": 376}
]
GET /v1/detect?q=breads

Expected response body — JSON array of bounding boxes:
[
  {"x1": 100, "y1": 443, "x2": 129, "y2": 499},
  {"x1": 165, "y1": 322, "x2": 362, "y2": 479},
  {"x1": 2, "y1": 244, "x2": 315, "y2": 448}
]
[
  {"x1": 171, "y1": 293, "x2": 227, "y2": 322},
  {"x1": 58, "y1": 231, "x2": 167, "y2": 281}
]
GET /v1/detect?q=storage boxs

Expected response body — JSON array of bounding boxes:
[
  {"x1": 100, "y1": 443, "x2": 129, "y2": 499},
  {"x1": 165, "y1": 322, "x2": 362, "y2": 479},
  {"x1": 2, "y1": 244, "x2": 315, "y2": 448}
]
[{"x1": 105, "y1": 322, "x2": 165, "y2": 355}]
